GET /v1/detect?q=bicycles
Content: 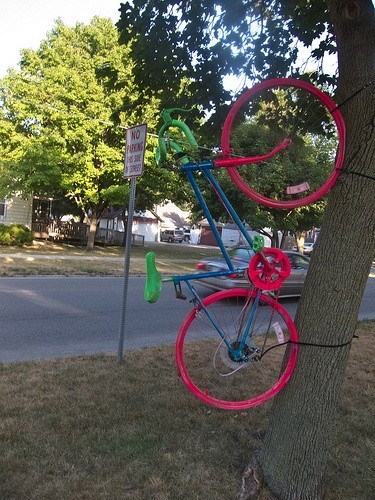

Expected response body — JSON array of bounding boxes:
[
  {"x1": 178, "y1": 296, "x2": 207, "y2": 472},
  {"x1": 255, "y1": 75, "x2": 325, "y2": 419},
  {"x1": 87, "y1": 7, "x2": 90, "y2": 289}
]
[{"x1": 145, "y1": 80, "x2": 348, "y2": 410}]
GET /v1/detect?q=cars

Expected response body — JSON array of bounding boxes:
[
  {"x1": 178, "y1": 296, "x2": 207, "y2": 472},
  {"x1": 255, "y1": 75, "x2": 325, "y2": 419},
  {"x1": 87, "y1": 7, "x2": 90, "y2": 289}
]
[
  {"x1": 294, "y1": 243, "x2": 315, "y2": 258},
  {"x1": 197, "y1": 247, "x2": 314, "y2": 307}
]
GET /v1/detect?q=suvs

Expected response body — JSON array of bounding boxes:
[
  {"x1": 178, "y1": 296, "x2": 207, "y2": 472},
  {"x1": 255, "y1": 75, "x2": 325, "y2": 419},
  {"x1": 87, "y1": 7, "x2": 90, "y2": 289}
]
[{"x1": 161, "y1": 229, "x2": 184, "y2": 243}]
